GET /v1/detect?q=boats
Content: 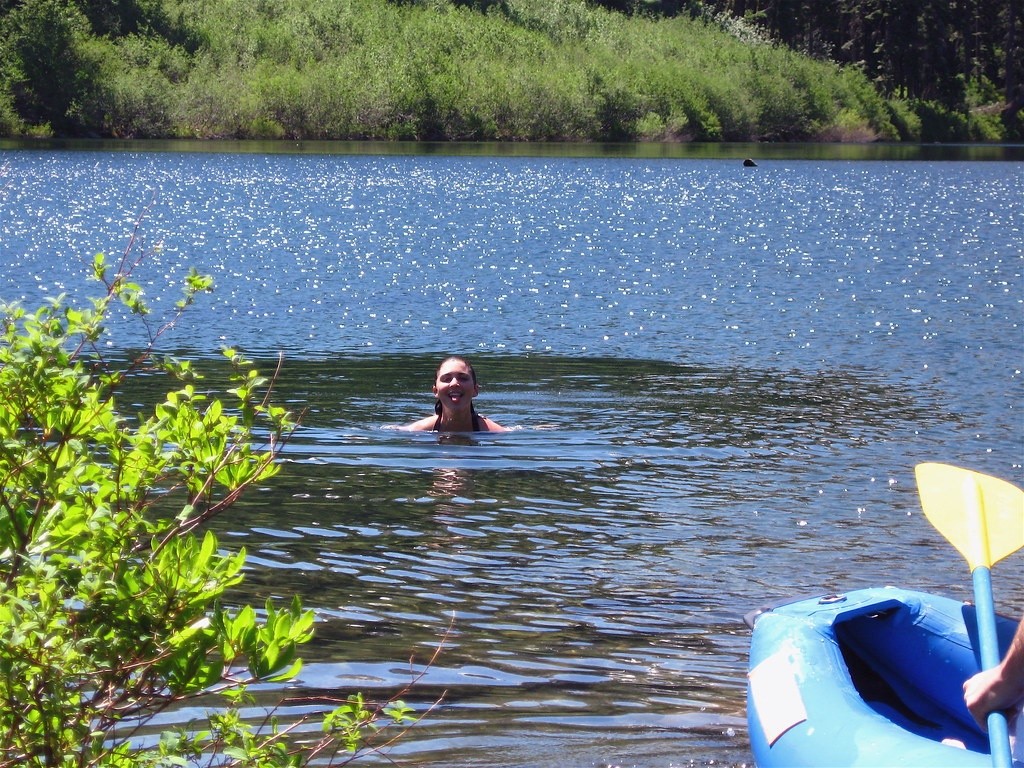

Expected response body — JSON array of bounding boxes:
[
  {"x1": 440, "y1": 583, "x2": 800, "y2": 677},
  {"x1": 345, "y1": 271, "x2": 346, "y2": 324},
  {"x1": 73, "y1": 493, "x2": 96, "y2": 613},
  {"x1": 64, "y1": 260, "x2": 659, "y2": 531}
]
[{"x1": 747, "y1": 586, "x2": 1024, "y2": 767}]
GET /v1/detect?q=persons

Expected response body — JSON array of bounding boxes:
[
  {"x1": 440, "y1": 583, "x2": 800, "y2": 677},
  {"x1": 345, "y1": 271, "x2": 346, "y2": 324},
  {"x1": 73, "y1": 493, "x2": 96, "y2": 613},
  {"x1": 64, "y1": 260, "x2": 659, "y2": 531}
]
[
  {"x1": 963, "y1": 611, "x2": 1024, "y2": 730},
  {"x1": 399, "y1": 358, "x2": 502, "y2": 435}
]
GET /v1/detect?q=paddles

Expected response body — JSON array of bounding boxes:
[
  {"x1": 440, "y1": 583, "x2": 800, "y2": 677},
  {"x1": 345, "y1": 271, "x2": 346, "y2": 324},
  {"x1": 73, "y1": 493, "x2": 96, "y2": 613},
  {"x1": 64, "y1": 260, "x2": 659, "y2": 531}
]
[{"x1": 914, "y1": 459, "x2": 1024, "y2": 768}]
